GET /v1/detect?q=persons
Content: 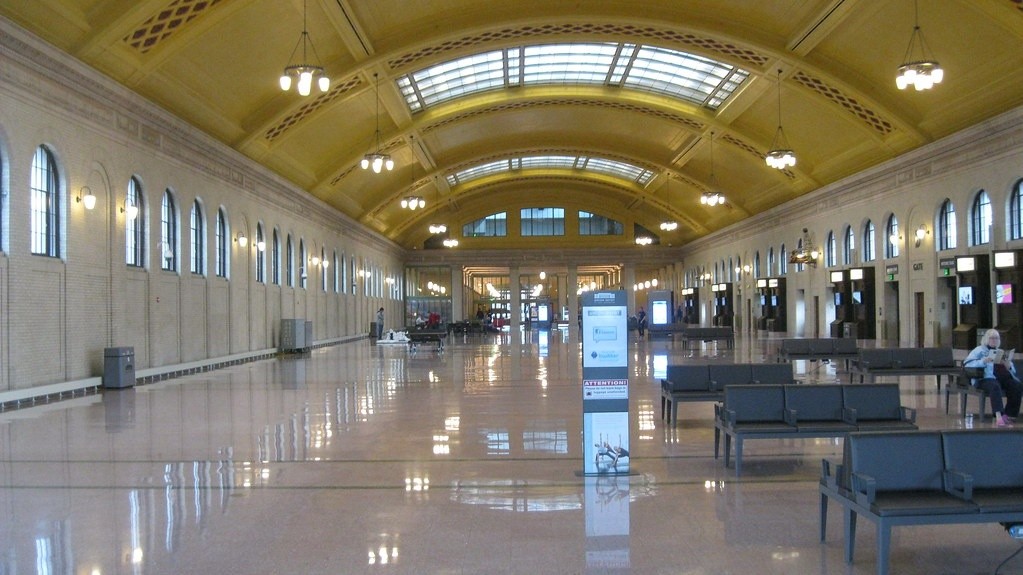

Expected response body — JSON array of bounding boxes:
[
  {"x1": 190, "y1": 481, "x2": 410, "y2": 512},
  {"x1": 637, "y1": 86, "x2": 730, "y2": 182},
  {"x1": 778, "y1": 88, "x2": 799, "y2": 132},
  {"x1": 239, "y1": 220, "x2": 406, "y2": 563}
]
[
  {"x1": 678, "y1": 306, "x2": 683, "y2": 321},
  {"x1": 476, "y1": 308, "x2": 493, "y2": 324},
  {"x1": 636, "y1": 306, "x2": 646, "y2": 338},
  {"x1": 376, "y1": 307, "x2": 384, "y2": 340},
  {"x1": 415, "y1": 315, "x2": 426, "y2": 329},
  {"x1": 963, "y1": 328, "x2": 1023, "y2": 427},
  {"x1": 594, "y1": 432, "x2": 629, "y2": 466},
  {"x1": 427, "y1": 311, "x2": 440, "y2": 329}
]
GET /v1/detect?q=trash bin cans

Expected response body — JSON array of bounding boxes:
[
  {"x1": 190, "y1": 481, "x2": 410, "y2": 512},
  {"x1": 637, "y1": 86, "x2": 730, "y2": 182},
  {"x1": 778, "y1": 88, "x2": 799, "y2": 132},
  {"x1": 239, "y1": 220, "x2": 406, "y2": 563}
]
[
  {"x1": 370, "y1": 322, "x2": 379, "y2": 336},
  {"x1": 104, "y1": 347, "x2": 135, "y2": 389},
  {"x1": 843, "y1": 322, "x2": 859, "y2": 338},
  {"x1": 976, "y1": 329, "x2": 987, "y2": 347}
]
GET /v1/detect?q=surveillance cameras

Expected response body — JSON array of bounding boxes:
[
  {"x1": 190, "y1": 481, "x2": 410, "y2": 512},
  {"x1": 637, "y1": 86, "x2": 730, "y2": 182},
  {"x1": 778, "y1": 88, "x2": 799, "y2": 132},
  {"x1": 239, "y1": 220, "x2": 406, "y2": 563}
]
[{"x1": 302, "y1": 273, "x2": 307, "y2": 279}]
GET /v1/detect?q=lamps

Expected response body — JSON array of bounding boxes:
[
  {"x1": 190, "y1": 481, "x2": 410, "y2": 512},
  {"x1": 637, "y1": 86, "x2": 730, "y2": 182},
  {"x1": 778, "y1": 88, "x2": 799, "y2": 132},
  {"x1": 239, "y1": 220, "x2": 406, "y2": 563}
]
[
  {"x1": 636, "y1": 173, "x2": 678, "y2": 245},
  {"x1": 429, "y1": 176, "x2": 458, "y2": 247},
  {"x1": 76, "y1": 186, "x2": 394, "y2": 284},
  {"x1": 700, "y1": 132, "x2": 725, "y2": 205},
  {"x1": 362, "y1": 74, "x2": 394, "y2": 173},
  {"x1": 280, "y1": 0, "x2": 330, "y2": 96},
  {"x1": 765, "y1": 69, "x2": 795, "y2": 169},
  {"x1": 427, "y1": 267, "x2": 658, "y2": 298},
  {"x1": 896, "y1": 0, "x2": 944, "y2": 92},
  {"x1": 401, "y1": 136, "x2": 425, "y2": 210}
]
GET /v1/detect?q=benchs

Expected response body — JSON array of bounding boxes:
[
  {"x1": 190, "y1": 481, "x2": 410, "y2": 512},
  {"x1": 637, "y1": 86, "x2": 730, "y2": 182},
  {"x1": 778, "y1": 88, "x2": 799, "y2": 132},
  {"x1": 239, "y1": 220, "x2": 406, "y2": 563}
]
[
  {"x1": 850, "y1": 347, "x2": 961, "y2": 389},
  {"x1": 776, "y1": 338, "x2": 857, "y2": 371},
  {"x1": 943, "y1": 358, "x2": 1023, "y2": 421},
  {"x1": 714, "y1": 383, "x2": 919, "y2": 475},
  {"x1": 660, "y1": 364, "x2": 802, "y2": 428},
  {"x1": 681, "y1": 327, "x2": 734, "y2": 349},
  {"x1": 647, "y1": 322, "x2": 687, "y2": 341},
  {"x1": 818, "y1": 427, "x2": 1023, "y2": 575},
  {"x1": 408, "y1": 321, "x2": 489, "y2": 352}
]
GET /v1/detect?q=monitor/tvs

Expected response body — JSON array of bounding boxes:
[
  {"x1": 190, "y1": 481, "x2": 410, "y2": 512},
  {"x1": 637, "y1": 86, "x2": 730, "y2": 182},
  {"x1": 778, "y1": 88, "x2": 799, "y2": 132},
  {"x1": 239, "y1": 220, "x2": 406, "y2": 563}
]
[
  {"x1": 722, "y1": 297, "x2": 725, "y2": 305},
  {"x1": 715, "y1": 298, "x2": 718, "y2": 305},
  {"x1": 761, "y1": 296, "x2": 766, "y2": 305},
  {"x1": 853, "y1": 291, "x2": 863, "y2": 304},
  {"x1": 996, "y1": 284, "x2": 1014, "y2": 305},
  {"x1": 958, "y1": 286, "x2": 974, "y2": 304},
  {"x1": 835, "y1": 293, "x2": 841, "y2": 305},
  {"x1": 689, "y1": 299, "x2": 693, "y2": 306},
  {"x1": 771, "y1": 296, "x2": 778, "y2": 306}
]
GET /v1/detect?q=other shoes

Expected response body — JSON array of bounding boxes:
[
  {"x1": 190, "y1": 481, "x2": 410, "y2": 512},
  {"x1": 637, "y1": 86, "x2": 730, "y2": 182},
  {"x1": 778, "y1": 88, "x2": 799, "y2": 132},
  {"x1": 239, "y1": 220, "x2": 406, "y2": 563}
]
[
  {"x1": 1002, "y1": 414, "x2": 1013, "y2": 424},
  {"x1": 997, "y1": 419, "x2": 1006, "y2": 426}
]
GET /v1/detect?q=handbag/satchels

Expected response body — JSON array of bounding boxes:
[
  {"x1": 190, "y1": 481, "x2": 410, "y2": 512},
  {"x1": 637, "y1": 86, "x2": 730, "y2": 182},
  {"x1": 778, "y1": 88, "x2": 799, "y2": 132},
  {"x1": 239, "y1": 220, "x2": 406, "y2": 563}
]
[{"x1": 962, "y1": 359, "x2": 984, "y2": 378}]
